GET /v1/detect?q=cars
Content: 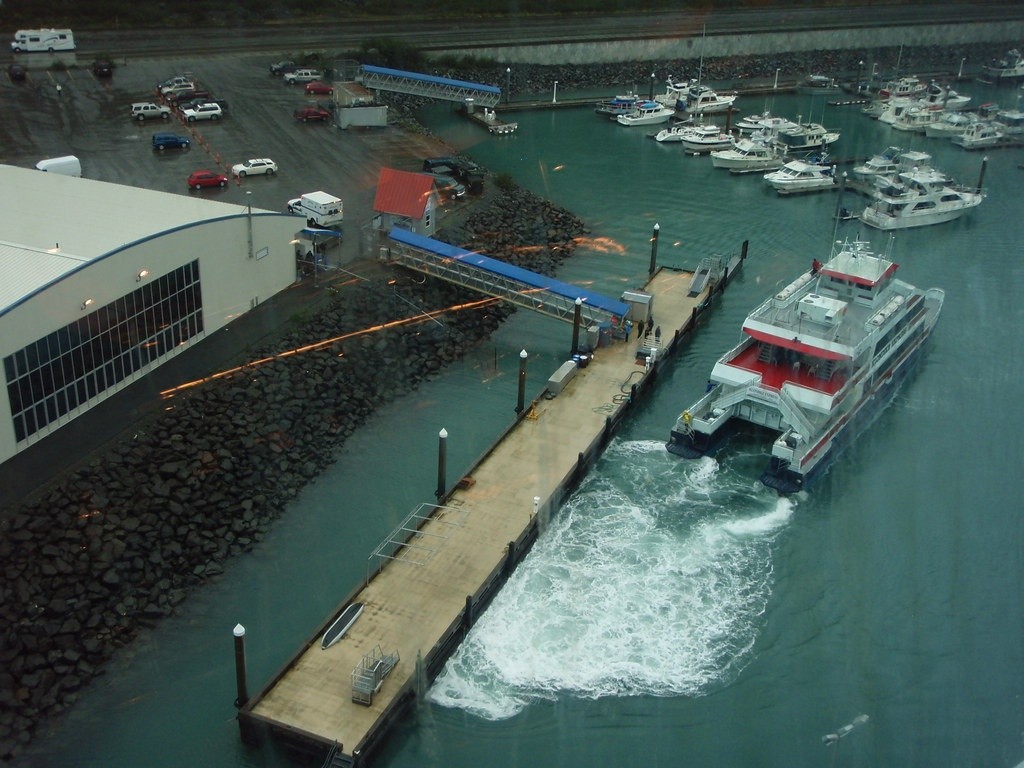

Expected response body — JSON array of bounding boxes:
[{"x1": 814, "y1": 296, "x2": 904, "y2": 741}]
[
  {"x1": 294, "y1": 105, "x2": 330, "y2": 122},
  {"x1": 158, "y1": 75, "x2": 228, "y2": 110},
  {"x1": 306, "y1": 80, "x2": 334, "y2": 94},
  {"x1": 8, "y1": 64, "x2": 25, "y2": 79},
  {"x1": 92, "y1": 59, "x2": 113, "y2": 78}
]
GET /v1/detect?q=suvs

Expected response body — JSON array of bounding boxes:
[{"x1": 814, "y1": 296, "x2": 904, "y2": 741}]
[
  {"x1": 189, "y1": 170, "x2": 229, "y2": 190},
  {"x1": 269, "y1": 60, "x2": 298, "y2": 76},
  {"x1": 182, "y1": 103, "x2": 223, "y2": 123},
  {"x1": 131, "y1": 102, "x2": 172, "y2": 121},
  {"x1": 231, "y1": 158, "x2": 278, "y2": 177},
  {"x1": 152, "y1": 132, "x2": 192, "y2": 151},
  {"x1": 283, "y1": 68, "x2": 323, "y2": 85},
  {"x1": 425, "y1": 172, "x2": 467, "y2": 200}
]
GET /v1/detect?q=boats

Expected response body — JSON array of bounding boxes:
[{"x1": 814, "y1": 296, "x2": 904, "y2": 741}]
[
  {"x1": 665, "y1": 207, "x2": 954, "y2": 498},
  {"x1": 594, "y1": 23, "x2": 1023, "y2": 231}
]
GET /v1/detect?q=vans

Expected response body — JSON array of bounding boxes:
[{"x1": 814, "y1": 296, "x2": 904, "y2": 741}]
[
  {"x1": 423, "y1": 157, "x2": 453, "y2": 175},
  {"x1": 36, "y1": 155, "x2": 81, "y2": 178}
]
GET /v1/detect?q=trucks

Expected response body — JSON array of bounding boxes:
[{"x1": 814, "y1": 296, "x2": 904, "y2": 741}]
[
  {"x1": 10, "y1": 27, "x2": 77, "y2": 51},
  {"x1": 287, "y1": 191, "x2": 344, "y2": 230}
]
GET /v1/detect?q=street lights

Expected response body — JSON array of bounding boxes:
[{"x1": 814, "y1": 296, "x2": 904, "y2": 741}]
[
  {"x1": 516, "y1": 349, "x2": 529, "y2": 415},
  {"x1": 648, "y1": 222, "x2": 661, "y2": 273},
  {"x1": 648, "y1": 72, "x2": 656, "y2": 103},
  {"x1": 436, "y1": 427, "x2": 452, "y2": 500},
  {"x1": 506, "y1": 67, "x2": 511, "y2": 103},
  {"x1": 571, "y1": 297, "x2": 582, "y2": 354},
  {"x1": 232, "y1": 624, "x2": 249, "y2": 710},
  {"x1": 857, "y1": 60, "x2": 864, "y2": 92}
]
